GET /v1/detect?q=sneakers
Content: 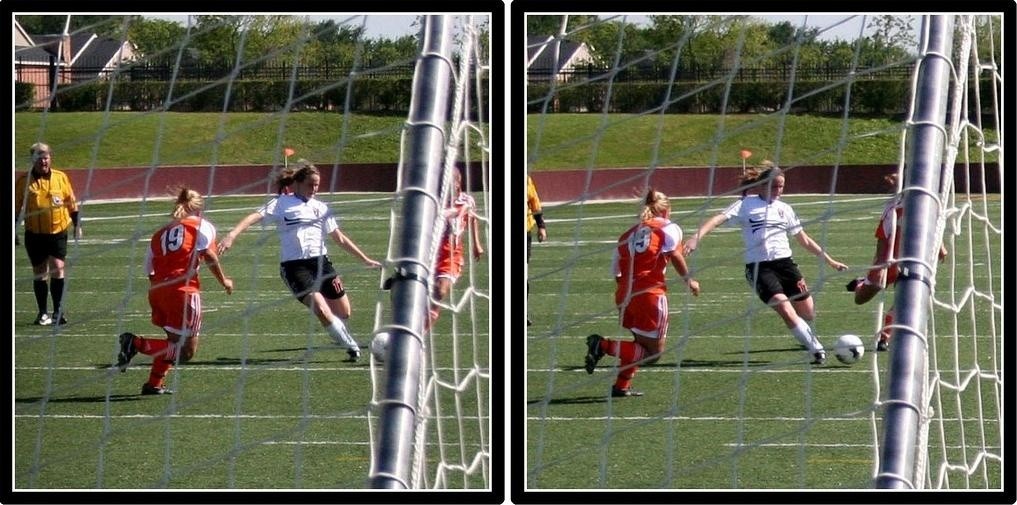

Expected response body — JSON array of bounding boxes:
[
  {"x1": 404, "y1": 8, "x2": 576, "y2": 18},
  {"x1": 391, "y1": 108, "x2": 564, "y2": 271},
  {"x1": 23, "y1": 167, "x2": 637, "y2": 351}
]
[
  {"x1": 611, "y1": 385, "x2": 643, "y2": 397},
  {"x1": 118, "y1": 333, "x2": 138, "y2": 372},
  {"x1": 142, "y1": 382, "x2": 173, "y2": 394},
  {"x1": 53, "y1": 313, "x2": 68, "y2": 325},
  {"x1": 814, "y1": 352, "x2": 826, "y2": 364},
  {"x1": 346, "y1": 346, "x2": 360, "y2": 362},
  {"x1": 35, "y1": 313, "x2": 51, "y2": 325},
  {"x1": 877, "y1": 339, "x2": 889, "y2": 351},
  {"x1": 584, "y1": 334, "x2": 605, "y2": 375},
  {"x1": 845, "y1": 278, "x2": 867, "y2": 291}
]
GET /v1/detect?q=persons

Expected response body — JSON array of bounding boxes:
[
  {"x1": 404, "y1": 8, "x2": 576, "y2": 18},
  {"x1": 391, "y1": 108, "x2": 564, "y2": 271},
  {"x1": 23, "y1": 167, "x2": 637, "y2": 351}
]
[
  {"x1": 14, "y1": 141, "x2": 83, "y2": 327},
  {"x1": 215, "y1": 156, "x2": 382, "y2": 364},
  {"x1": 680, "y1": 157, "x2": 849, "y2": 367},
  {"x1": 583, "y1": 183, "x2": 701, "y2": 398},
  {"x1": 843, "y1": 171, "x2": 947, "y2": 353},
  {"x1": 116, "y1": 179, "x2": 234, "y2": 396},
  {"x1": 424, "y1": 164, "x2": 484, "y2": 335},
  {"x1": 525, "y1": 173, "x2": 547, "y2": 326}
]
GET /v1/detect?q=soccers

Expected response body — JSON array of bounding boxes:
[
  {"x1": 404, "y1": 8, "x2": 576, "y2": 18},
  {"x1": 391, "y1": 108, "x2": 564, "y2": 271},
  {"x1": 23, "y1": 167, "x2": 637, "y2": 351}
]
[
  {"x1": 373, "y1": 333, "x2": 389, "y2": 360},
  {"x1": 834, "y1": 334, "x2": 864, "y2": 364}
]
